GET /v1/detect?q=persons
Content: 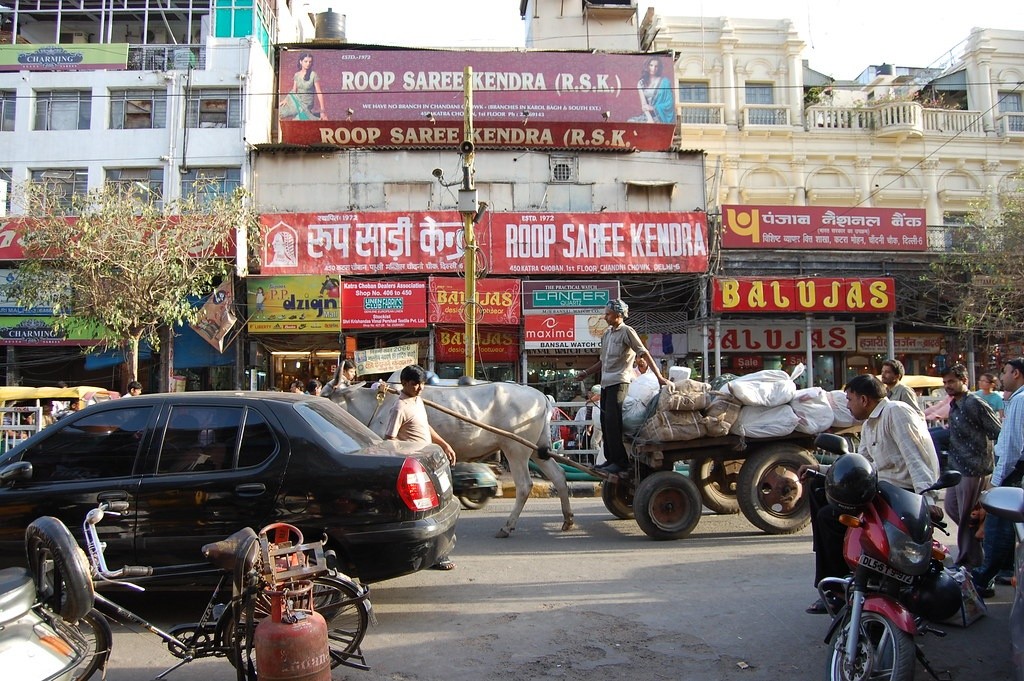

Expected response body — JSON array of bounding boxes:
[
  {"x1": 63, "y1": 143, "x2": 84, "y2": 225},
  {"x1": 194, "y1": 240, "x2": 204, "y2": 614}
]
[
  {"x1": 543, "y1": 385, "x2": 556, "y2": 403},
  {"x1": 290, "y1": 359, "x2": 356, "y2": 397},
  {"x1": 121, "y1": 381, "x2": 143, "y2": 398},
  {"x1": 573, "y1": 299, "x2": 675, "y2": 473},
  {"x1": 318, "y1": 275, "x2": 339, "y2": 297},
  {"x1": 3, "y1": 398, "x2": 79, "y2": 438},
  {"x1": 797, "y1": 357, "x2": 1024, "y2": 615},
  {"x1": 248, "y1": 287, "x2": 264, "y2": 318},
  {"x1": 384, "y1": 365, "x2": 456, "y2": 570}
]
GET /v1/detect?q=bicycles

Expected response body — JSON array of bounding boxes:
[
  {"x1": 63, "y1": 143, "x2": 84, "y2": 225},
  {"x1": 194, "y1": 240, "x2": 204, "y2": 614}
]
[{"x1": 72, "y1": 500, "x2": 378, "y2": 680}]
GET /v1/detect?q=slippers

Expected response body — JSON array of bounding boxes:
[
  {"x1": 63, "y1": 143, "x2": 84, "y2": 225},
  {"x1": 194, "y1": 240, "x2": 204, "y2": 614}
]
[{"x1": 806, "y1": 589, "x2": 846, "y2": 613}]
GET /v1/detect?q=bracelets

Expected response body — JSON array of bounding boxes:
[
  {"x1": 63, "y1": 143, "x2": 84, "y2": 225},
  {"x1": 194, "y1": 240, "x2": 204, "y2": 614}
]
[{"x1": 582, "y1": 370, "x2": 587, "y2": 376}]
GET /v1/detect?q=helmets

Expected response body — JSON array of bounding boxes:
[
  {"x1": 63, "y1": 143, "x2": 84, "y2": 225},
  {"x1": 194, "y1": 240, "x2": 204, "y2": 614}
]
[{"x1": 825, "y1": 451, "x2": 879, "y2": 513}]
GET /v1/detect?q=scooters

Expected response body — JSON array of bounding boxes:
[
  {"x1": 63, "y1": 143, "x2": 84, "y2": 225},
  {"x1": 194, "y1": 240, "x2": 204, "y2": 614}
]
[
  {"x1": 449, "y1": 461, "x2": 497, "y2": 511},
  {"x1": 0, "y1": 517, "x2": 92, "y2": 681}
]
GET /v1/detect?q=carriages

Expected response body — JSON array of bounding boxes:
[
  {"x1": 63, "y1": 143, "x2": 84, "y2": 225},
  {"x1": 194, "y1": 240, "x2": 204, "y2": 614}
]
[{"x1": 321, "y1": 360, "x2": 864, "y2": 538}]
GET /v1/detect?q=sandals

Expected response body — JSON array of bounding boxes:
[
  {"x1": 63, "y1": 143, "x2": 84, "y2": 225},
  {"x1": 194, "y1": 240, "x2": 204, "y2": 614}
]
[{"x1": 433, "y1": 557, "x2": 455, "y2": 571}]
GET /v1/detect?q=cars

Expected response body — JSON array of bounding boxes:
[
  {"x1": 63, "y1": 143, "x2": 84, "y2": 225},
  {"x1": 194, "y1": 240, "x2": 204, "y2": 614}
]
[{"x1": 0, "y1": 391, "x2": 461, "y2": 624}]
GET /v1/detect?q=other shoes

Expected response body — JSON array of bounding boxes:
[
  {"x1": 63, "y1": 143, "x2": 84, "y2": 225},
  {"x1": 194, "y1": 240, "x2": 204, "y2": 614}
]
[
  {"x1": 999, "y1": 572, "x2": 1015, "y2": 586},
  {"x1": 972, "y1": 572, "x2": 996, "y2": 596}
]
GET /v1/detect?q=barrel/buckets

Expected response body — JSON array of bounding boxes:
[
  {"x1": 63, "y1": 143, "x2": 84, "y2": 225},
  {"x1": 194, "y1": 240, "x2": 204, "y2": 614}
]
[{"x1": 315, "y1": 12, "x2": 345, "y2": 39}]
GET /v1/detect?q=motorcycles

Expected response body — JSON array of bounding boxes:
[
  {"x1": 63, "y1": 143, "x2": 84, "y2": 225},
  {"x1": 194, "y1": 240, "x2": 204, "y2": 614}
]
[
  {"x1": 803, "y1": 432, "x2": 962, "y2": 680},
  {"x1": 978, "y1": 487, "x2": 1024, "y2": 678}
]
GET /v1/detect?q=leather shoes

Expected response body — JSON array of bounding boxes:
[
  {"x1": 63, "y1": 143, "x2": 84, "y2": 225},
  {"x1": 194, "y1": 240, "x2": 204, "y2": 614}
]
[{"x1": 594, "y1": 461, "x2": 629, "y2": 474}]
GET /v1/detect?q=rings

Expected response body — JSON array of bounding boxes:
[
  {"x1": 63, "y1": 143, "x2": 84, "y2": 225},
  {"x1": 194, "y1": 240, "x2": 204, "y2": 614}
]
[{"x1": 794, "y1": 480, "x2": 799, "y2": 482}]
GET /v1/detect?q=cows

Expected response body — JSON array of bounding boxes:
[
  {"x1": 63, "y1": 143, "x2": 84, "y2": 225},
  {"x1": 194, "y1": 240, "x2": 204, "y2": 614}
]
[{"x1": 318, "y1": 361, "x2": 574, "y2": 538}]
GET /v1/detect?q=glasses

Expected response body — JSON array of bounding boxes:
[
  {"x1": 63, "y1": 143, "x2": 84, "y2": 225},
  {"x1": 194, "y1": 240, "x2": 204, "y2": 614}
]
[{"x1": 978, "y1": 380, "x2": 994, "y2": 384}]
